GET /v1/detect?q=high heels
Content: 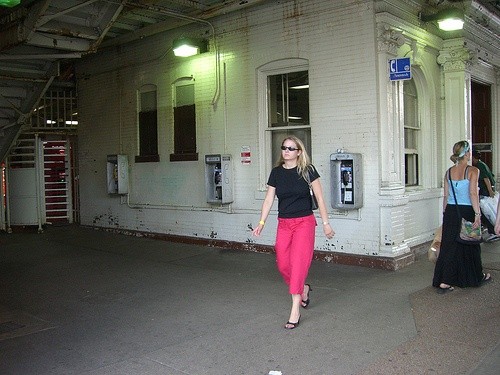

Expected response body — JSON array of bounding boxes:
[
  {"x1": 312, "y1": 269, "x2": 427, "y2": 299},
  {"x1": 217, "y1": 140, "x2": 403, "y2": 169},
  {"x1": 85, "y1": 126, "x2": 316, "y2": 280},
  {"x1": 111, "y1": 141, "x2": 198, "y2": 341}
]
[
  {"x1": 285, "y1": 315, "x2": 301, "y2": 329},
  {"x1": 301, "y1": 284, "x2": 312, "y2": 308},
  {"x1": 439, "y1": 284, "x2": 454, "y2": 293},
  {"x1": 481, "y1": 273, "x2": 490, "y2": 281}
]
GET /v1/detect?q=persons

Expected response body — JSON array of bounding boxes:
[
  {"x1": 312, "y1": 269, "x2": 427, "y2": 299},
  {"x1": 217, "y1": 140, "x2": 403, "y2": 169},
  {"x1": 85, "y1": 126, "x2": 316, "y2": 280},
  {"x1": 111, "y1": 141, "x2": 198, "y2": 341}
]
[
  {"x1": 432, "y1": 141, "x2": 491, "y2": 293},
  {"x1": 471, "y1": 150, "x2": 495, "y2": 236},
  {"x1": 250, "y1": 136, "x2": 335, "y2": 329},
  {"x1": 493, "y1": 195, "x2": 500, "y2": 235}
]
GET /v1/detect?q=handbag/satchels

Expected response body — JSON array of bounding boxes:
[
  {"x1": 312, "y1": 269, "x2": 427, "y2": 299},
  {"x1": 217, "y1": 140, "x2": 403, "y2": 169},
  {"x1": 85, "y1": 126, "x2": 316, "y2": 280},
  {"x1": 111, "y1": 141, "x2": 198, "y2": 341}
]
[
  {"x1": 460, "y1": 218, "x2": 481, "y2": 242},
  {"x1": 428, "y1": 226, "x2": 443, "y2": 262},
  {"x1": 307, "y1": 170, "x2": 318, "y2": 209}
]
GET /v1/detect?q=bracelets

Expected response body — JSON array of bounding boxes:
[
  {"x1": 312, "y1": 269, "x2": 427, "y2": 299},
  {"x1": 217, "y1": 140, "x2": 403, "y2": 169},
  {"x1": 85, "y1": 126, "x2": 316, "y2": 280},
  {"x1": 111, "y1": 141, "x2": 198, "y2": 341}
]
[
  {"x1": 475, "y1": 212, "x2": 481, "y2": 216},
  {"x1": 322, "y1": 221, "x2": 329, "y2": 225},
  {"x1": 258, "y1": 220, "x2": 265, "y2": 225}
]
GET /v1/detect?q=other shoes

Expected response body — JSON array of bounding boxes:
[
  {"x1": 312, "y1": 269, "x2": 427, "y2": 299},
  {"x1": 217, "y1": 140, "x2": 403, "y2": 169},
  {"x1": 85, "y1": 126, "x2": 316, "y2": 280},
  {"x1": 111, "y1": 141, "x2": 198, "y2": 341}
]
[{"x1": 487, "y1": 234, "x2": 500, "y2": 242}]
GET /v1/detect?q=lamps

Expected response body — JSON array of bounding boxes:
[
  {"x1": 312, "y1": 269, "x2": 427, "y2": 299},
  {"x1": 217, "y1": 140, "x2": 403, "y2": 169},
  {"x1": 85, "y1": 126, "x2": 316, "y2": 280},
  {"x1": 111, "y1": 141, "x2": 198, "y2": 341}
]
[
  {"x1": 420, "y1": 8, "x2": 464, "y2": 30},
  {"x1": 172, "y1": 37, "x2": 208, "y2": 57}
]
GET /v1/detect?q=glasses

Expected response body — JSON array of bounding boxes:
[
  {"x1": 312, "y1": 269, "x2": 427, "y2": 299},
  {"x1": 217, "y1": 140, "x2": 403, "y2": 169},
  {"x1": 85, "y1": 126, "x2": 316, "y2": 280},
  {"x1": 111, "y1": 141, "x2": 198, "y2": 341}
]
[{"x1": 281, "y1": 146, "x2": 299, "y2": 151}]
